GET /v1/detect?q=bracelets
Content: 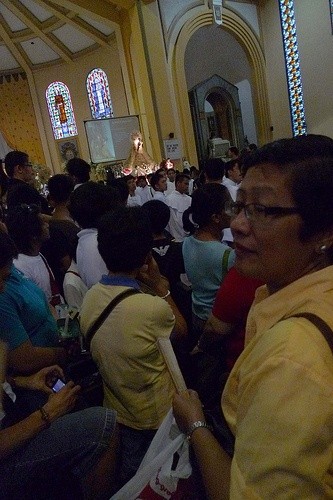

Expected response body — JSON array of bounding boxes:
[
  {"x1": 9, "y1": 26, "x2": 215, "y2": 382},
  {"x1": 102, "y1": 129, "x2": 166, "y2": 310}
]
[
  {"x1": 186, "y1": 421, "x2": 212, "y2": 439},
  {"x1": 39, "y1": 407, "x2": 52, "y2": 424}
]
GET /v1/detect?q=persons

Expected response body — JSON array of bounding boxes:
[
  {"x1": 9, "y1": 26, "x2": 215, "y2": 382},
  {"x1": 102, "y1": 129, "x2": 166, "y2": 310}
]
[
  {"x1": 0, "y1": 141, "x2": 266, "y2": 500},
  {"x1": 172, "y1": 134, "x2": 333, "y2": 500}
]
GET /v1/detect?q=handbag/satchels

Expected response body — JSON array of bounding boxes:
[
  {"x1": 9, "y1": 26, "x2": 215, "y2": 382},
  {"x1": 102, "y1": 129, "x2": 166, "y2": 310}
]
[{"x1": 68, "y1": 350, "x2": 104, "y2": 393}]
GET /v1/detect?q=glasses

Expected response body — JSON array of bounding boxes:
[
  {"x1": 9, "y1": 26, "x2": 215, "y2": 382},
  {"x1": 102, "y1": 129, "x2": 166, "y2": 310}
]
[
  {"x1": 223, "y1": 199, "x2": 300, "y2": 220},
  {"x1": 21, "y1": 203, "x2": 31, "y2": 213},
  {"x1": 23, "y1": 164, "x2": 33, "y2": 168}
]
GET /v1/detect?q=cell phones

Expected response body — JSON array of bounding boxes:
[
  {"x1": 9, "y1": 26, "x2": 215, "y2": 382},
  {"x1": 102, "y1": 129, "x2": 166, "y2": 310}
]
[{"x1": 46, "y1": 375, "x2": 66, "y2": 393}]
